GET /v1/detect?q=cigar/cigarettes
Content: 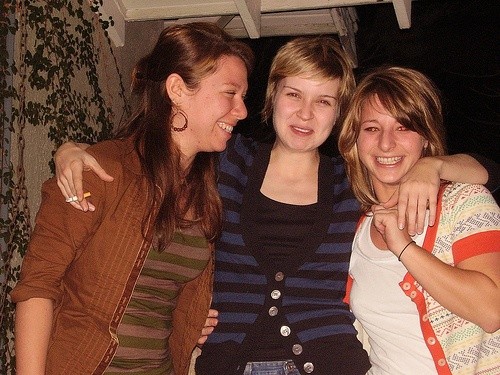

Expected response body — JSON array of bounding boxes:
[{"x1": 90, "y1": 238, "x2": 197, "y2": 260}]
[{"x1": 66, "y1": 192, "x2": 91, "y2": 202}]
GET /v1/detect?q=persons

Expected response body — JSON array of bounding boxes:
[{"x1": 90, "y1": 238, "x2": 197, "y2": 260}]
[
  {"x1": 53, "y1": 37, "x2": 488, "y2": 375},
  {"x1": 10, "y1": 22, "x2": 247, "y2": 375},
  {"x1": 337, "y1": 67, "x2": 500, "y2": 375}
]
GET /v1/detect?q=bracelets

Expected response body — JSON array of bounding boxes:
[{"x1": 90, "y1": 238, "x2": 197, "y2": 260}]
[{"x1": 398, "y1": 241, "x2": 416, "y2": 261}]
